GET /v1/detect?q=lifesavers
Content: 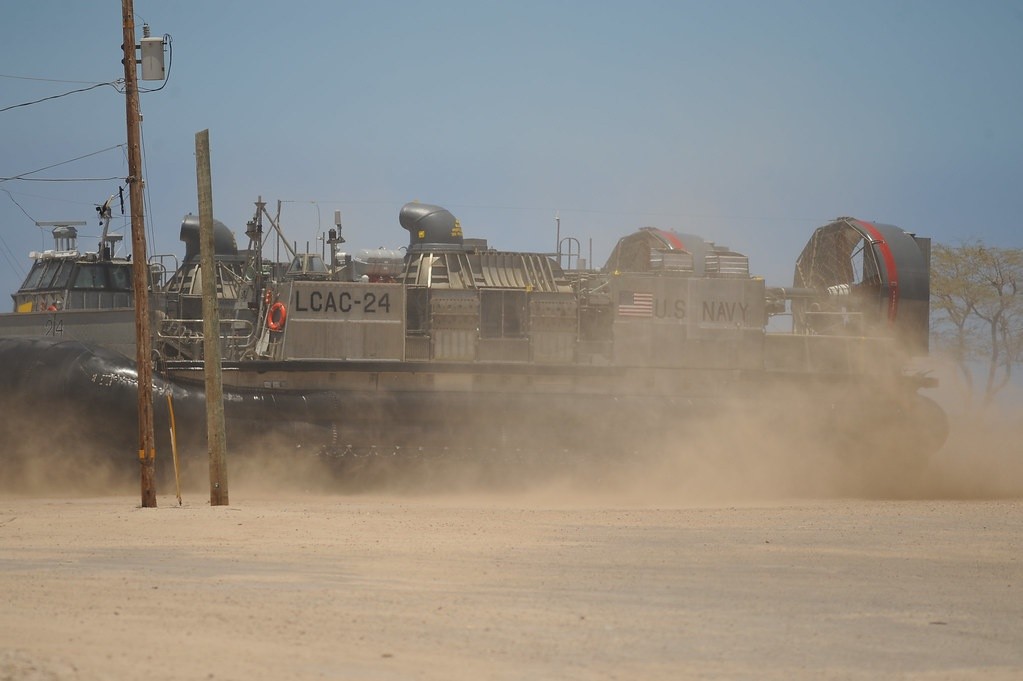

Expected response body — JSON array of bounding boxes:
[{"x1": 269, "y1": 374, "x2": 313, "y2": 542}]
[{"x1": 268, "y1": 303, "x2": 286, "y2": 330}]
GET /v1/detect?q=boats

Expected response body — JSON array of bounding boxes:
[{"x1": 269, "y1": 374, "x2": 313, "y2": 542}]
[{"x1": 1, "y1": 198, "x2": 949, "y2": 500}]
